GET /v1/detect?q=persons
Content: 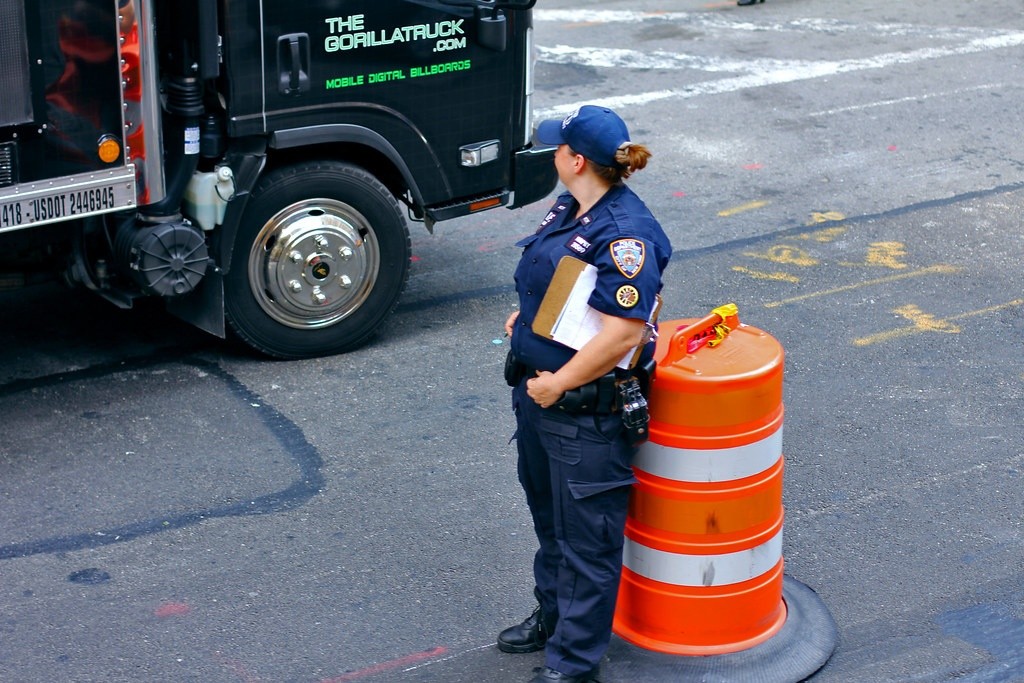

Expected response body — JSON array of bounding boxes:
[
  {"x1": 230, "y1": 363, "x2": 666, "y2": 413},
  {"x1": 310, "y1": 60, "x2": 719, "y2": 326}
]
[{"x1": 498, "y1": 105, "x2": 672, "y2": 683}]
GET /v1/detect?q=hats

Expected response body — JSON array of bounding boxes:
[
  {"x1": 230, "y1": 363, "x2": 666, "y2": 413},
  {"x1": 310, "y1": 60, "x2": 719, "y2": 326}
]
[{"x1": 537, "y1": 105, "x2": 629, "y2": 169}]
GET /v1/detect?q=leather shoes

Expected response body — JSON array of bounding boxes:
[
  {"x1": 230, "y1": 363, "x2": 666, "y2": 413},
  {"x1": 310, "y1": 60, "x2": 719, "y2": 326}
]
[
  {"x1": 527, "y1": 665, "x2": 597, "y2": 683},
  {"x1": 498, "y1": 610, "x2": 552, "y2": 652}
]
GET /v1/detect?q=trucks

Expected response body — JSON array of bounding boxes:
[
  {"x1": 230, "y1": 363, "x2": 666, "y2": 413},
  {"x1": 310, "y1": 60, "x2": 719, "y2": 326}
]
[{"x1": 0, "y1": 0, "x2": 559, "y2": 361}]
